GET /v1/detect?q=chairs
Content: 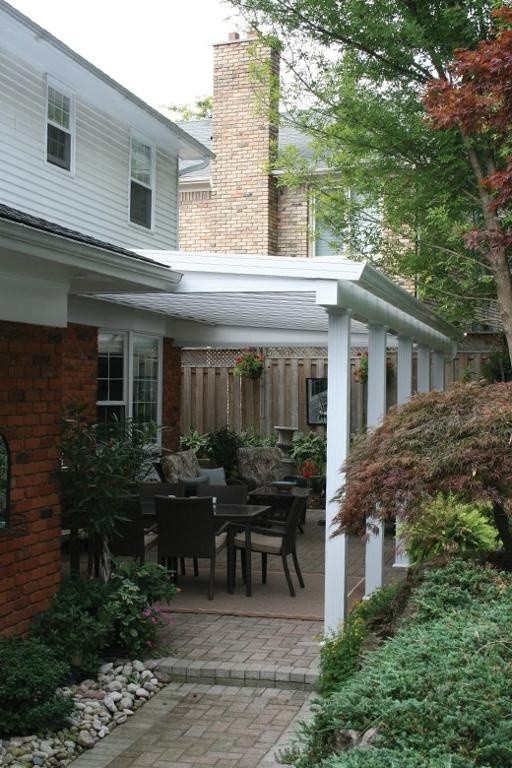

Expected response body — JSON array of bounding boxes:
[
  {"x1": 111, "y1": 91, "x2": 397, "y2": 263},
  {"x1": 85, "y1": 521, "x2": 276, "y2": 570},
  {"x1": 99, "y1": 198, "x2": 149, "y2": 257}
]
[{"x1": 94, "y1": 447, "x2": 309, "y2": 599}]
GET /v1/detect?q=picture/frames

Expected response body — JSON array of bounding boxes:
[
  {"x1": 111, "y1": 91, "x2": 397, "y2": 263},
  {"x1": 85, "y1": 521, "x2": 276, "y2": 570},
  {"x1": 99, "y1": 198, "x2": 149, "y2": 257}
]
[{"x1": 306, "y1": 378, "x2": 327, "y2": 425}]
